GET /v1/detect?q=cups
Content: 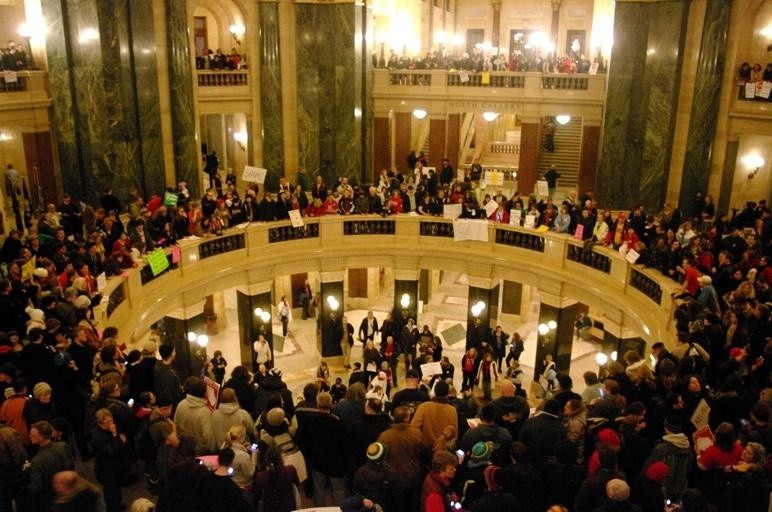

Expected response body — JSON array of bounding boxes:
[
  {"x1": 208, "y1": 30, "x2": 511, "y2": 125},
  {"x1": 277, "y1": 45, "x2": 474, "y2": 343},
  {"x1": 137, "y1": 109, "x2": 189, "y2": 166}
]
[
  {"x1": 111, "y1": 387, "x2": 120, "y2": 391},
  {"x1": 102, "y1": 418, "x2": 114, "y2": 424}
]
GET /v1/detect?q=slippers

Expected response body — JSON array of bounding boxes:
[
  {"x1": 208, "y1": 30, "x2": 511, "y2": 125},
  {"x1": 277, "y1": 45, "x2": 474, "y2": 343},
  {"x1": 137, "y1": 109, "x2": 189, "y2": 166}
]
[{"x1": 273, "y1": 432, "x2": 308, "y2": 484}]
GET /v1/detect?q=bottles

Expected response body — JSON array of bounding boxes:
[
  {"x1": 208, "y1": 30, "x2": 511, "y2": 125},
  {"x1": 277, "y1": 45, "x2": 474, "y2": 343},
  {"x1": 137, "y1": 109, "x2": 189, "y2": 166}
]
[
  {"x1": 607, "y1": 479, "x2": 631, "y2": 500},
  {"x1": 697, "y1": 275, "x2": 712, "y2": 284},
  {"x1": 143, "y1": 341, "x2": 156, "y2": 353},
  {"x1": 483, "y1": 466, "x2": 504, "y2": 491},
  {"x1": 25, "y1": 307, "x2": 44, "y2": 322},
  {"x1": 599, "y1": 428, "x2": 620, "y2": 450},
  {"x1": 470, "y1": 441, "x2": 494, "y2": 462},
  {"x1": 33, "y1": 268, "x2": 48, "y2": 277},
  {"x1": 33, "y1": 382, "x2": 51, "y2": 398},
  {"x1": 556, "y1": 372, "x2": 571, "y2": 388},
  {"x1": 268, "y1": 368, "x2": 283, "y2": 377},
  {"x1": 267, "y1": 408, "x2": 285, "y2": 426},
  {"x1": 406, "y1": 369, "x2": 421, "y2": 378},
  {"x1": 647, "y1": 461, "x2": 670, "y2": 482},
  {"x1": 366, "y1": 442, "x2": 388, "y2": 465},
  {"x1": 662, "y1": 414, "x2": 682, "y2": 433},
  {"x1": 479, "y1": 404, "x2": 495, "y2": 421}
]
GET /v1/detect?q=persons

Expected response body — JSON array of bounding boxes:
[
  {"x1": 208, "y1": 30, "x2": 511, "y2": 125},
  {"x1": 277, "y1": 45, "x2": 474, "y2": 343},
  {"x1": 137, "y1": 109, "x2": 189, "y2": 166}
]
[
  {"x1": 337, "y1": 316, "x2": 354, "y2": 369},
  {"x1": 257, "y1": 150, "x2": 597, "y2": 266},
  {"x1": 277, "y1": 296, "x2": 292, "y2": 338},
  {"x1": 581, "y1": 191, "x2": 771, "y2": 512},
  {"x1": 574, "y1": 313, "x2": 592, "y2": 340},
  {"x1": 298, "y1": 279, "x2": 313, "y2": 320},
  {"x1": 193, "y1": 48, "x2": 249, "y2": 85},
  {"x1": 357, "y1": 309, "x2": 557, "y2": 401},
  {"x1": 254, "y1": 335, "x2": 272, "y2": 374},
  {"x1": 0, "y1": 40, "x2": 37, "y2": 92},
  {"x1": 544, "y1": 119, "x2": 556, "y2": 153},
  {"x1": 373, "y1": 31, "x2": 609, "y2": 90},
  {"x1": 763, "y1": 64, "x2": 772, "y2": 101},
  {"x1": 2, "y1": 150, "x2": 257, "y2": 511},
  {"x1": 250, "y1": 361, "x2": 586, "y2": 511},
  {"x1": 750, "y1": 63, "x2": 764, "y2": 83},
  {"x1": 739, "y1": 62, "x2": 751, "y2": 97}
]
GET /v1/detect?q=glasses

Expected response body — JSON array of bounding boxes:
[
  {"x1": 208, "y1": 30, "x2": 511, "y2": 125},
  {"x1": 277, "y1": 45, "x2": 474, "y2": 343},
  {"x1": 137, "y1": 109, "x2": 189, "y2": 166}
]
[
  {"x1": 401, "y1": 298, "x2": 410, "y2": 320},
  {"x1": 483, "y1": 112, "x2": 497, "y2": 122},
  {"x1": 470, "y1": 301, "x2": 485, "y2": 327},
  {"x1": 596, "y1": 352, "x2": 608, "y2": 376},
  {"x1": 748, "y1": 157, "x2": 764, "y2": 179},
  {"x1": 230, "y1": 25, "x2": 245, "y2": 45},
  {"x1": 195, "y1": 336, "x2": 208, "y2": 365},
  {"x1": 556, "y1": 115, "x2": 571, "y2": 125},
  {"x1": 413, "y1": 110, "x2": 426, "y2": 120},
  {"x1": 329, "y1": 300, "x2": 338, "y2": 322},
  {"x1": 611, "y1": 351, "x2": 618, "y2": 361},
  {"x1": 538, "y1": 320, "x2": 557, "y2": 348},
  {"x1": 262, "y1": 312, "x2": 270, "y2": 328},
  {"x1": 234, "y1": 132, "x2": 246, "y2": 153}
]
